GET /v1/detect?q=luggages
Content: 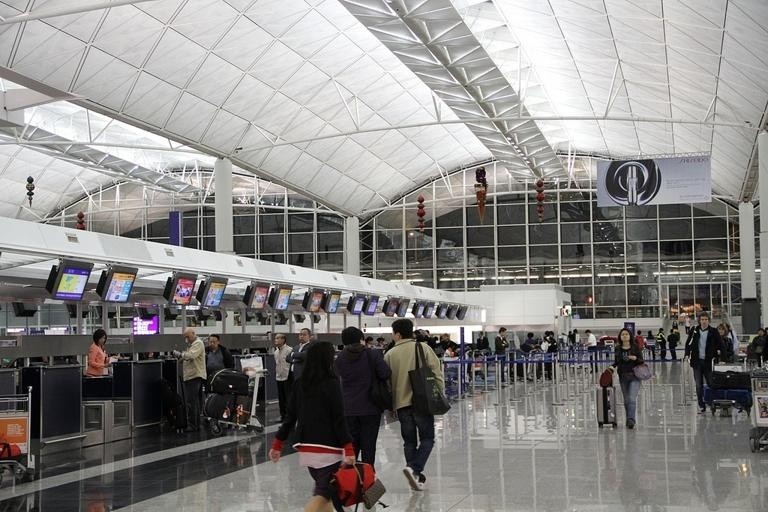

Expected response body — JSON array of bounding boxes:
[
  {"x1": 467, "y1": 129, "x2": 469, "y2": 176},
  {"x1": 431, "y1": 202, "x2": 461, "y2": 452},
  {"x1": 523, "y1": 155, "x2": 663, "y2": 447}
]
[
  {"x1": 596, "y1": 386, "x2": 617, "y2": 428},
  {"x1": 702, "y1": 384, "x2": 748, "y2": 403}
]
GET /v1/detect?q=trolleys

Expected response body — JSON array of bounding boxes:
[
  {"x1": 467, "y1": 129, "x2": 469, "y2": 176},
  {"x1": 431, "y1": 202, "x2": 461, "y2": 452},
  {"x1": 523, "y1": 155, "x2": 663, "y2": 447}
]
[
  {"x1": 746, "y1": 357, "x2": 768, "y2": 452},
  {"x1": 710, "y1": 356, "x2": 748, "y2": 416},
  {"x1": 0, "y1": 386, "x2": 36, "y2": 485},
  {"x1": 202, "y1": 366, "x2": 271, "y2": 436}
]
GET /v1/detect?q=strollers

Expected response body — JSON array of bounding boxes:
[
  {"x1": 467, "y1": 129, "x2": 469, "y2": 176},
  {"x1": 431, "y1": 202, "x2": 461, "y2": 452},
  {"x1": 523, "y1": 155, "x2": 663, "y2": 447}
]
[{"x1": 468, "y1": 350, "x2": 488, "y2": 380}]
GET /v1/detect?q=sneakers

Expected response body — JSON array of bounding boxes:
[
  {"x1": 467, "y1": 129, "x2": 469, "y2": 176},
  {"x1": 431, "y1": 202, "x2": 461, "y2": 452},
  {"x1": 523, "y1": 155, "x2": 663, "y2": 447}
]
[{"x1": 403, "y1": 467, "x2": 424, "y2": 491}]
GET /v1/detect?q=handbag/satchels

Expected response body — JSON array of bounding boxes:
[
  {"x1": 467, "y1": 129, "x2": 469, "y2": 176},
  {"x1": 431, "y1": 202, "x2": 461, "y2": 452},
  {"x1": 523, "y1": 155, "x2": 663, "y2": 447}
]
[
  {"x1": 327, "y1": 462, "x2": 375, "y2": 512},
  {"x1": 709, "y1": 370, "x2": 750, "y2": 390},
  {"x1": 599, "y1": 368, "x2": 613, "y2": 387},
  {"x1": 367, "y1": 348, "x2": 393, "y2": 411},
  {"x1": 353, "y1": 461, "x2": 386, "y2": 510},
  {"x1": 408, "y1": 343, "x2": 451, "y2": 416},
  {"x1": 632, "y1": 363, "x2": 651, "y2": 380},
  {"x1": 202, "y1": 367, "x2": 253, "y2": 424}
]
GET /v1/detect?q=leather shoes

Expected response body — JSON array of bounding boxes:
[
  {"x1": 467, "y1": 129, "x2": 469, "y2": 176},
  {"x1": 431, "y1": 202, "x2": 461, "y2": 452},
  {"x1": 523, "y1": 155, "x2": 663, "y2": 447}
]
[{"x1": 627, "y1": 420, "x2": 634, "y2": 429}]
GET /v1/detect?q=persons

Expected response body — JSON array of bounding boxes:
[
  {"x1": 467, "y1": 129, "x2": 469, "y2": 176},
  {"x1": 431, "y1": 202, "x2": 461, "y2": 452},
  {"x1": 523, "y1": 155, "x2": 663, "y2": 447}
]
[
  {"x1": 615, "y1": 312, "x2": 768, "y2": 428},
  {"x1": 86, "y1": 328, "x2": 113, "y2": 377},
  {"x1": 205, "y1": 334, "x2": 235, "y2": 398},
  {"x1": 172, "y1": 327, "x2": 208, "y2": 432},
  {"x1": 269, "y1": 318, "x2": 457, "y2": 512},
  {"x1": 475, "y1": 323, "x2": 600, "y2": 379}
]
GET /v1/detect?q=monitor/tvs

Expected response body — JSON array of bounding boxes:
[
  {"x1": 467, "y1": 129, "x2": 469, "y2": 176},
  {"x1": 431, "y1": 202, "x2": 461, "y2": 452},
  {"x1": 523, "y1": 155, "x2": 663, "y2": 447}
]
[
  {"x1": 301, "y1": 288, "x2": 324, "y2": 313},
  {"x1": 96, "y1": 306, "x2": 116, "y2": 319},
  {"x1": 257, "y1": 313, "x2": 268, "y2": 321},
  {"x1": 196, "y1": 309, "x2": 212, "y2": 321},
  {"x1": 309, "y1": 314, "x2": 321, "y2": 323},
  {"x1": 136, "y1": 307, "x2": 157, "y2": 320},
  {"x1": 213, "y1": 310, "x2": 228, "y2": 321},
  {"x1": 395, "y1": 298, "x2": 410, "y2": 318},
  {"x1": 294, "y1": 314, "x2": 306, "y2": 323},
  {"x1": 12, "y1": 301, "x2": 37, "y2": 317},
  {"x1": 346, "y1": 293, "x2": 365, "y2": 315},
  {"x1": 241, "y1": 280, "x2": 270, "y2": 311},
  {"x1": 435, "y1": 302, "x2": 448, "y2": 319},
  {"x1": 456, "y1": 305, "x2": 468, "y2": 320},
  {"x1": 267, "y1": 284, "x2": 293, "y2": 311},
  {"x1": 246, "y1": 312, "x2": 255, "y2": 321},
  {"x1": 67, "y1": 304, "x2": 89, "y2": 319},
  {"x1": 162, "y1": 272, "x2": 198, "y2": 306},
  {"x1": 94, "y1": 265, "x2": 138, "y2": 303},
  {"x1": 195, "y1": 275, "x2": 228, "y2": 308},
  {"x1": 275, "y1": 314, "x2": 288, "y2": 325},
  {"x1": 362, "y1": 295, "x2": 380, "y2": 316},
  {"x1": 382, "y1": 297, "x2": 400, "y2": 318},
  {"x1": 45, "y1": 258, "x2": 94, "y2": 301},
  {"x1": 446, "y1": 303, "x2": 459, "y2": 320},
  {"x1": 411, "y1": 300, "x2": 425, "y2": 319},
  {"x1": 422, "y1": 302, "x2": 437, "y2": 319},
  {"x1": 164, "y1": 307, "x2": 178, "y2": 321},
  {"x1": 322, "y1": 289, "x2": 342, "y2": 314}
]
